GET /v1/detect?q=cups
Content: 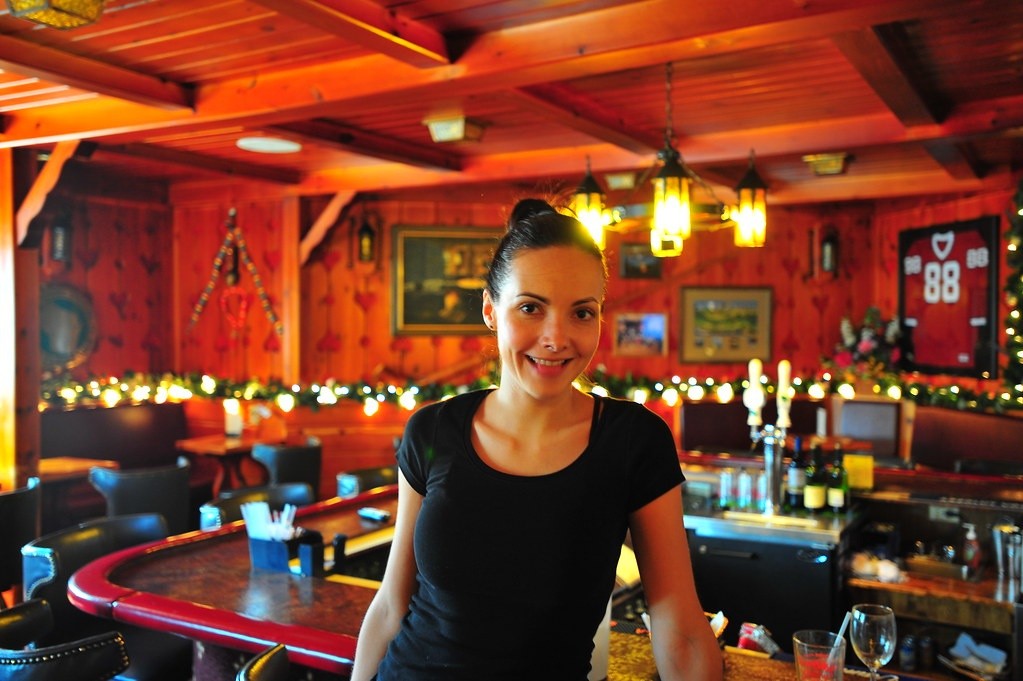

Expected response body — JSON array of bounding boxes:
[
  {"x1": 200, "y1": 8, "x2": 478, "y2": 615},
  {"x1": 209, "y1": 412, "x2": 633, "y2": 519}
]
[{"x1": 792, "y1": 629, "x2": 846, "y2": 681}]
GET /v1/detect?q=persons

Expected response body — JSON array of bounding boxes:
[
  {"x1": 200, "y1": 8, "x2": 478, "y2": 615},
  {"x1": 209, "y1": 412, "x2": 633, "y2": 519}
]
[{"x1": 348, "y1": 197, "x2": 724, "y2": 680}]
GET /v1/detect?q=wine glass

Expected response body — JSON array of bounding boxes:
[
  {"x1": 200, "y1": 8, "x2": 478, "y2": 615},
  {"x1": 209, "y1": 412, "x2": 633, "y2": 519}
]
[{"x1": 849, "y1": 604, "x2": 897, "y2": 681}]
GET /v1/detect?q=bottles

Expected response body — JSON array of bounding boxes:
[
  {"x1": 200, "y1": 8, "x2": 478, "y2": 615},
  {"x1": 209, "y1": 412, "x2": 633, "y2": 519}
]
[{"x1": 783, "y1": 435, "x2": 851, "y2": 513}]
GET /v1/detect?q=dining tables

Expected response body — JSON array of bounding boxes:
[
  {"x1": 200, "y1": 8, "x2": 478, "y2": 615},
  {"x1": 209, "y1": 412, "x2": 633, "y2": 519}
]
[{"x1": 179, "y1": 437, "x2": 287, "y2": 498}]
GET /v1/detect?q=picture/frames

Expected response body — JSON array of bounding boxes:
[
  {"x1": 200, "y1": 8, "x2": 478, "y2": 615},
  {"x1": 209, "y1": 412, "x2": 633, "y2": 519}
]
[
  {"x1": 390, "y1": 227, "x2": 504, "y2": 334},
  {"x1": 680, "y1": 284, "x2": 773, "y2": 365},
  {"x1": 612, "y1": 242, "x2": 668, "y2": 356},
  {"x1": 896, "y1": 213, "x2": 998, "y2": 380}
]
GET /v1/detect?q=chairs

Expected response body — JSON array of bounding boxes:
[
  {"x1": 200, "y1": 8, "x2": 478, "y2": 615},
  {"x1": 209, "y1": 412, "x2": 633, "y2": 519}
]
[
  {"x1": 337, "y1": 464, "x2": 399, "y2": 498},
  {"x1": 0, "y1": 436, "x2": 322, "y2": 681}
]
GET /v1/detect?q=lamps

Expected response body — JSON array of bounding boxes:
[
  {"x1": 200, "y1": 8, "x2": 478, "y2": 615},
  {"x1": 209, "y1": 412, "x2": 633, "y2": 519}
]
[
  {"x1": 7, "y1": 0, "x2": 102, "y2": 31},
  {"x1": 425, "y1": 117, "x2": 488, "y2": 142},
  {"x1": 745, "y1": 359, "x2": 795, "y2": 512},
  {"x1": 802, "y1": 152, "x2": 853, "y2": 177},
  {"x1": 566, "y1": 62, "x2": 768, "y2": 256}
]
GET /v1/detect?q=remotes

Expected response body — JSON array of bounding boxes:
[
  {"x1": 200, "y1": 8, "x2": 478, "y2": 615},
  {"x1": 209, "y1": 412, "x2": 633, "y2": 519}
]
[{"x1": 357, "y1": 507, "x2": 391, "y2": 520}]
